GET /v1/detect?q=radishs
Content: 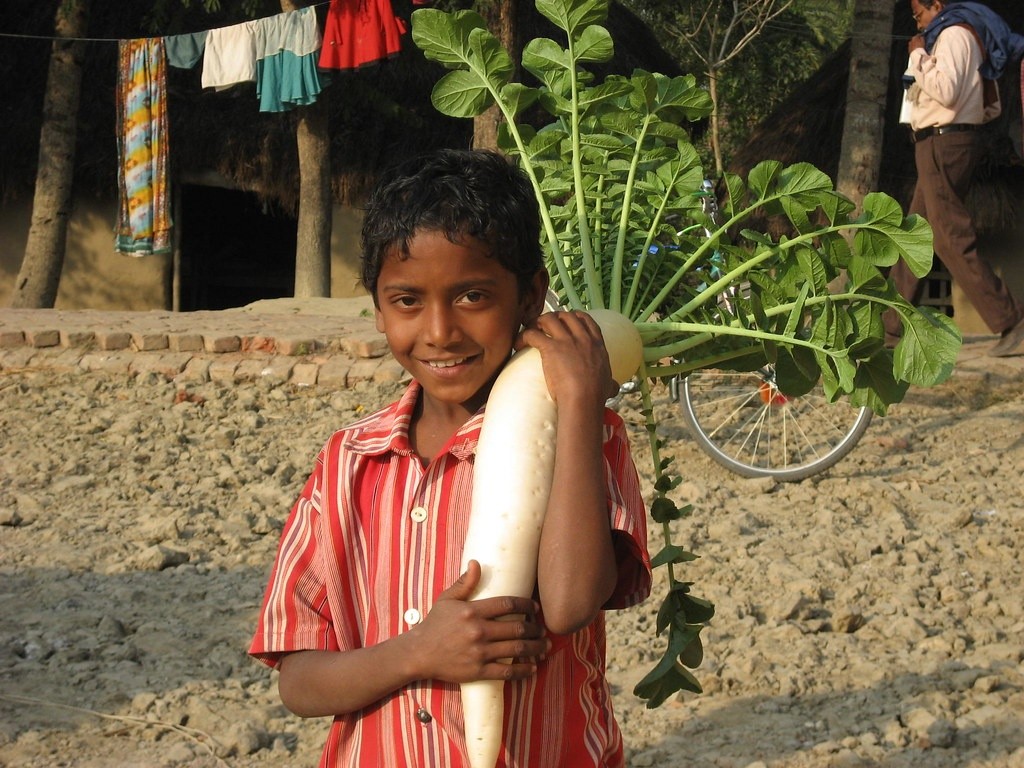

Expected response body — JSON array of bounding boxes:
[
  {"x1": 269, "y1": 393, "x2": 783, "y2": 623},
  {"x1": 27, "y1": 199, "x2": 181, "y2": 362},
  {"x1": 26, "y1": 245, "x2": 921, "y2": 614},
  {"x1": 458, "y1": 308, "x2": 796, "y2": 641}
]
[{"x1": 449, "y1": 309, "x2": 643, "y2": 768}]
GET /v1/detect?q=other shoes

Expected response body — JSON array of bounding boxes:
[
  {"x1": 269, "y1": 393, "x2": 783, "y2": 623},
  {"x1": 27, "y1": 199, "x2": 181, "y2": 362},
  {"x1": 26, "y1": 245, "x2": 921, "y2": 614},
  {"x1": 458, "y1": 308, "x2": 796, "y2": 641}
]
[{"x1": 988, "y1": 318, "x2": 1024, "y2": 356}]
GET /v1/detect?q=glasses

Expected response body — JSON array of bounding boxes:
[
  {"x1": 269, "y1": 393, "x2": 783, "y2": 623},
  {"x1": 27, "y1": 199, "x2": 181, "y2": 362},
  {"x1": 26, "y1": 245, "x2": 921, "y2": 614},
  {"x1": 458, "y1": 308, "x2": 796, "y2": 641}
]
[{"x1": 912, "y1": 0, "x2": 947, "y2": 22}]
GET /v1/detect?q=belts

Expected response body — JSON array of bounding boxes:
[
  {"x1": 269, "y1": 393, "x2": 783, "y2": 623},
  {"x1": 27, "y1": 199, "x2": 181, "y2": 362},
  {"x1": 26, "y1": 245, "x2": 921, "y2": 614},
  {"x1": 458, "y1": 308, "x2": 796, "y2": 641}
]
[{"x1": 911, "y1": 124, "x2": 980, "y2": 143}]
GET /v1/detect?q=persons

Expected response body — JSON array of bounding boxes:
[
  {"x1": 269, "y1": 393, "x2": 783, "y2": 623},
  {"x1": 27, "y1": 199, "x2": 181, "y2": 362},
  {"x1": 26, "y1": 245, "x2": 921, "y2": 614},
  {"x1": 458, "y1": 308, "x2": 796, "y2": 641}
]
[
  {"x1": 880, "y1": 0, "x2": 1024, "y2": 356},
  {"x1": 251, "y1": 151, "x2": 652, "y2": 768}
]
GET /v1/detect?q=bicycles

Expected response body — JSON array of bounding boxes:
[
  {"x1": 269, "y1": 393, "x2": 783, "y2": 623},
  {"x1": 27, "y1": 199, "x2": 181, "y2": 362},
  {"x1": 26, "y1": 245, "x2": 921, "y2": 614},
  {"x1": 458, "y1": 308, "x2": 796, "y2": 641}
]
[{"x1": 510, "y1": 181, "x2": 873, "y2": 482}]
